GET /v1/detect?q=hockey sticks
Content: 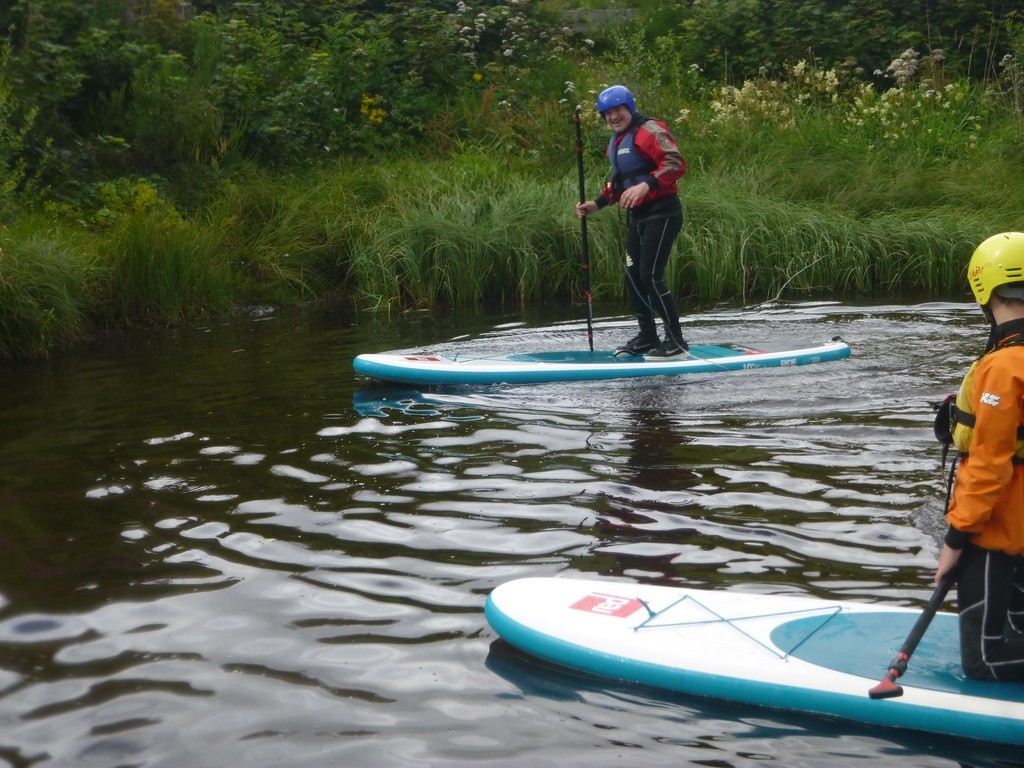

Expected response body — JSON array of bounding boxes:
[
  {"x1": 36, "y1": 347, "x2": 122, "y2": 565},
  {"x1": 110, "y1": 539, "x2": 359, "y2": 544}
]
[
  {"x1": 570, "y1": 102, "x2": 598, "y2": 350},
  {"x1": 868, "y1": 563, "x2": 957, "y2": 701}
]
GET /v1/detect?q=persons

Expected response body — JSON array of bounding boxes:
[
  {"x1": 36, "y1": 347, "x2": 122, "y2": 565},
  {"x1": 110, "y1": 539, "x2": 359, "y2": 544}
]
[
  {"x1": 935, "y1": 232, "x2": 1024, "y2": 683},
  {"x1": 576, "y1": 86, "x2": 691, "y2": 362}
]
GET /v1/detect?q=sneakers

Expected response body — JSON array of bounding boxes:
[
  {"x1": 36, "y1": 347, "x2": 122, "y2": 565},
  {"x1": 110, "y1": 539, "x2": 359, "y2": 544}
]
[
  {"x1": 615, "y1": 333, "x2": 663, "y2": 358},
  {"x1": 644, "y1": 338, "x2": 689, "y2": 360}
]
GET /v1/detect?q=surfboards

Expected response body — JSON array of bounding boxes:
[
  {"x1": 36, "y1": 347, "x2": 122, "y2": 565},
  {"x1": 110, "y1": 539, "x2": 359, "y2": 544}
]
[
  {"x1": 356, "y1": 337, "x2": 854, "y2": 388},
  {"x1": 483, "y1": 574, "x2": 1024, "y2": 745}
]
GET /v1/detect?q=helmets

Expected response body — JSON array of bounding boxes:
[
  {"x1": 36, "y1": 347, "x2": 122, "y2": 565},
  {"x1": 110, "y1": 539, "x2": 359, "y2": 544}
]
[
  {"x1": 597, "y1": 85, "x2": 636, "y2": 119},
  {"x1": 966, "y1": 231, "x2": 1024, "y2": 305}
]
